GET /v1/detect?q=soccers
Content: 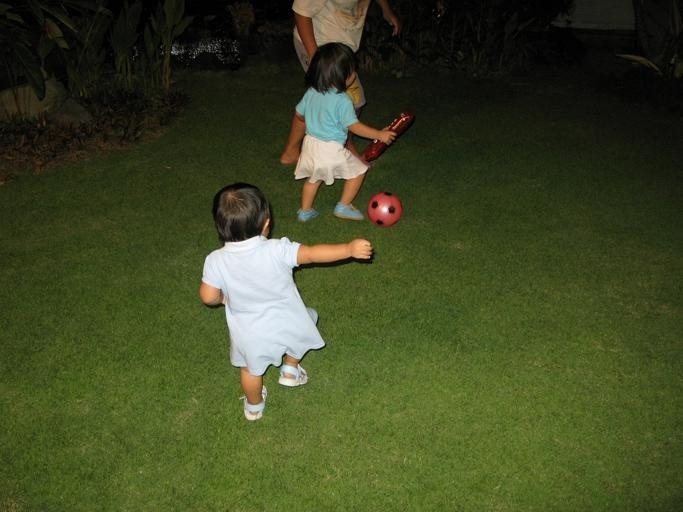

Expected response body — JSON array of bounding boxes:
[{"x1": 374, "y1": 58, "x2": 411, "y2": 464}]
[{"x1": 368, "y1": 192, "x2": 402, "y2": 227}]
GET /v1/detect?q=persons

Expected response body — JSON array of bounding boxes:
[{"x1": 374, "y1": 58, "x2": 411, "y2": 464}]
[
  {"x1": 279, "y1": 0, "x2": 404, "y2": 166},
  {"x1": 293, "y1": 41, "x2": 397, "y2": 224},
  {"x1": 196, "y1": 179, "x2": 375, "y2": 421}
]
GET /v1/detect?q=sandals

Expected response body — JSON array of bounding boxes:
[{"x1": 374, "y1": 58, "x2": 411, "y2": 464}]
[
  {"x1": 279, "y1": 153, "x2": 299, "y2": 168},
  {"x1": 333, "y1": 202, "x2": 363, "y2": 220},
  {"x1": 240, "y1": 385, "x2": 268, "y2": 421},
  {"x1": 297, "y1": 208, "x2": 319, "y2": 222},
  {"x1": 345, "y1": 142, "x2": 371, "y2": 169},
  {"x1": 278, "y1": 365, "x2": 308, "y2": 387}
]
